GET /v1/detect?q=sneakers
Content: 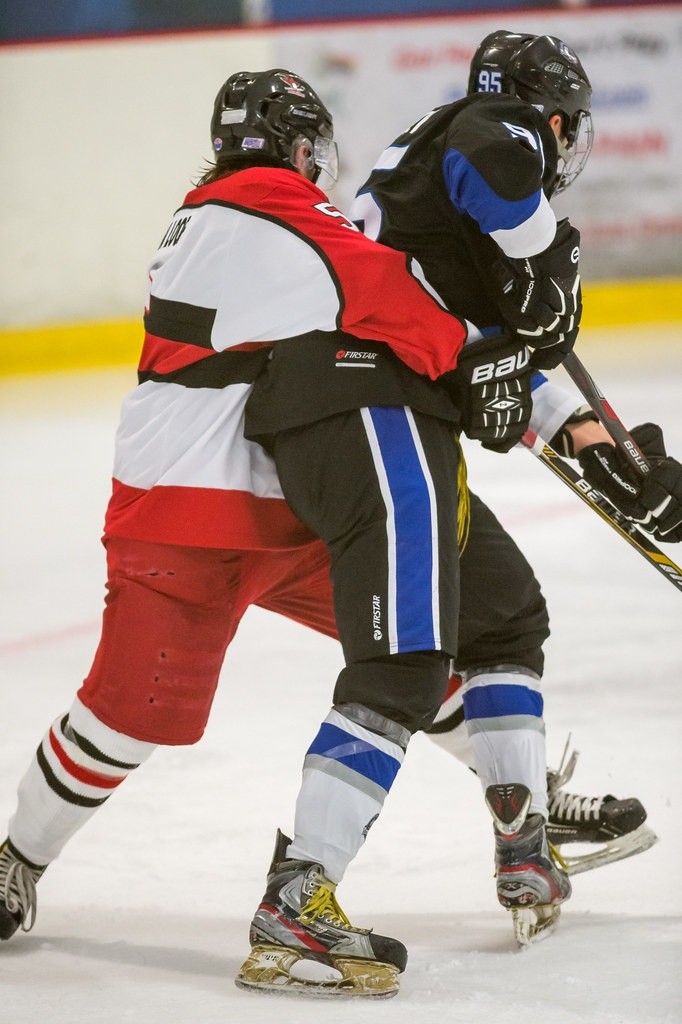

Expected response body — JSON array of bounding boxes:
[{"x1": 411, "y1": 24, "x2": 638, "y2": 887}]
[
  {"x1": 235, "y1": 828, "x2": 407, "y2": 1000},
  {"x1": 0, "y1": 836, "x2": 48, "y2": 941},
  {"x1": 544, "y1": 731, "x2": 658, "y2": 877},
  {"x1": 485, "y1": 783, "x2": 572, "y2": 948}
]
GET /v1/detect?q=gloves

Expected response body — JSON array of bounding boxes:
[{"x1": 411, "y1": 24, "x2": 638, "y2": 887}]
[
  {"x1": 505, "y1": 217, "x2": 583, "y2": 371},
  {"x1": 449, "y1": 315, "x2": 540, "y2": 454},
  {"x1": 577, "y1": 422, "x2": 682, "y2": 544}
]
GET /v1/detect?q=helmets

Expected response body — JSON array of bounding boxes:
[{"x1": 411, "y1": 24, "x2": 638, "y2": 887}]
[
  {"x1": 467, "y1": 29, "x2": 593, "y2": 121},
  {"x1": 209, "y1": 68, "x2": 334, "y2": 171}
]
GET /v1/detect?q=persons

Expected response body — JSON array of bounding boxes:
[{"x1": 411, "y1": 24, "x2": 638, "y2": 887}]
[
  {"x1": 236, "y1": 30, "x2": 682, "y2": 1000},
  {"x1": 0, "y1": 69, "x2": 659, "y2": 941}
]
